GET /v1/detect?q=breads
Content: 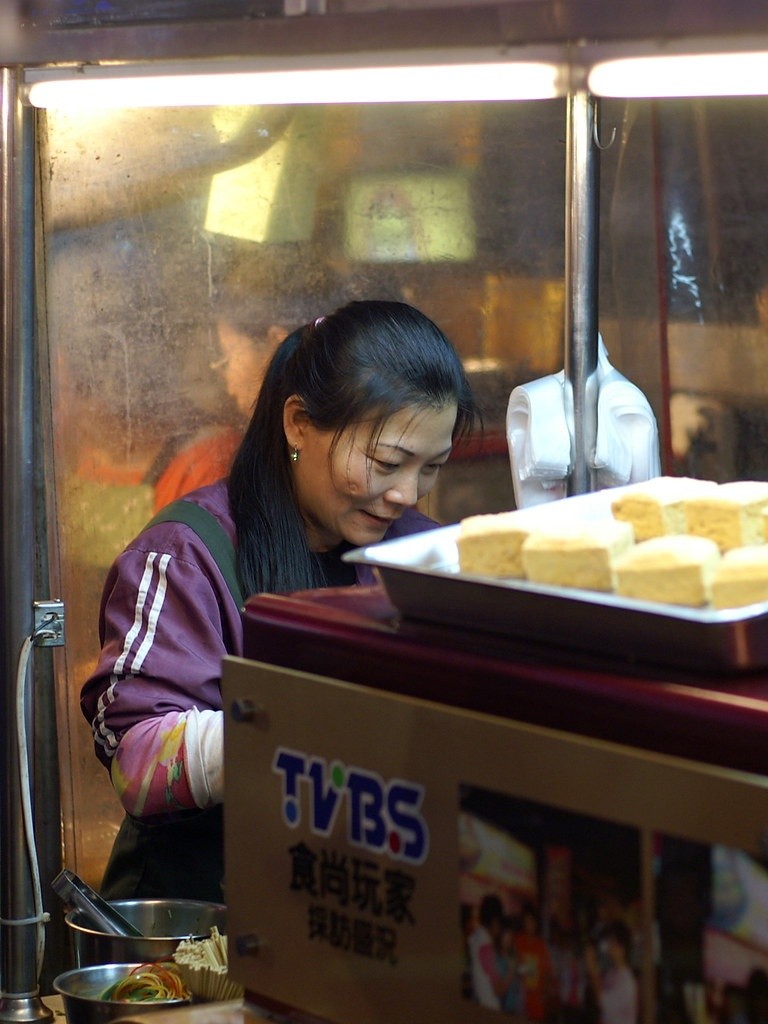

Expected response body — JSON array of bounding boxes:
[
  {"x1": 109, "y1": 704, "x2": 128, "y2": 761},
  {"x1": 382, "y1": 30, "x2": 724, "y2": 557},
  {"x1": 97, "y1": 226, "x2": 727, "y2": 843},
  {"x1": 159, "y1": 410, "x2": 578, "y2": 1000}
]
[{"x1": 455, "y1": 479, "x2": 768, "y2": 608}]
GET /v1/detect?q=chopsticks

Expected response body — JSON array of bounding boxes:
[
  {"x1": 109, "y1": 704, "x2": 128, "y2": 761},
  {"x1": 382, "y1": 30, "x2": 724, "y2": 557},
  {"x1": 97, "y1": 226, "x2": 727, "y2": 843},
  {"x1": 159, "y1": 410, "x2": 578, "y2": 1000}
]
[{"x1": 171, "y1": 925, "x2": 244, "y2": 1005}]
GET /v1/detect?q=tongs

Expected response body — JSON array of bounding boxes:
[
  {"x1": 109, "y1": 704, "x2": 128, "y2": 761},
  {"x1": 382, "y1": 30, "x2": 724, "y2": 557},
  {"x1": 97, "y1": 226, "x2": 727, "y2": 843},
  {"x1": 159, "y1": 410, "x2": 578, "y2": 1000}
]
[{"x1": 51, "y1": 868, "x2": 143, "y2": 937}]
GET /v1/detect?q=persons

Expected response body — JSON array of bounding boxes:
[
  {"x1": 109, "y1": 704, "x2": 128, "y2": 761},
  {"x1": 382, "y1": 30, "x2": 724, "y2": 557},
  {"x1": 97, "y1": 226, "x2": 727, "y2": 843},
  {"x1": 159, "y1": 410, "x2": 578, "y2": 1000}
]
[
  {"x1": 466, "y1": 892, "x2": 640, "y2": 1024},
  {"x1": 155, "y1": 252, "x2": 358, "y2": 510},
  {"x1": 60, "y1": 338, "x2": 218, "y2": 486},
  {"x1": 80, "y1": 301, "x2": 475, "y2": 901}
]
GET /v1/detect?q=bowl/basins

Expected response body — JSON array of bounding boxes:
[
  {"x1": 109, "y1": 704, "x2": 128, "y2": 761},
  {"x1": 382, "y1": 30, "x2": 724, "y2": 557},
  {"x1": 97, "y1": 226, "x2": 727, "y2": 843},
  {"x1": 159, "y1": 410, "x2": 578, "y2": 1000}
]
[
  {"x1": 53, "y1": 963, "x2": 191, "y2": 1024},
  {"x1": 65, "y1": 898, "x2": 225, "y2": 969}
]
